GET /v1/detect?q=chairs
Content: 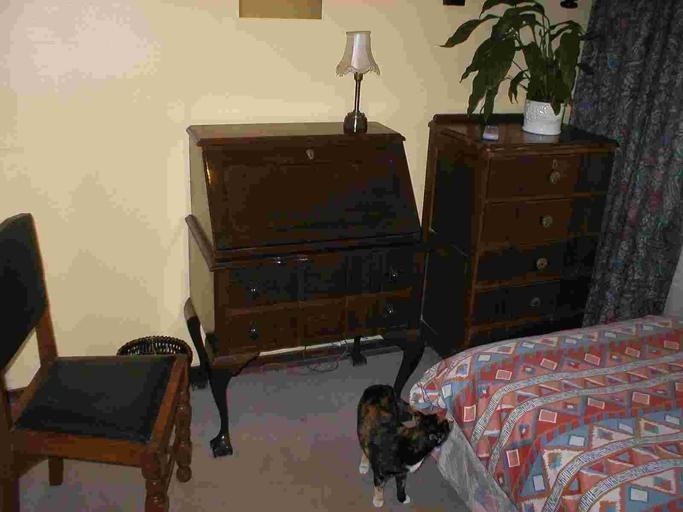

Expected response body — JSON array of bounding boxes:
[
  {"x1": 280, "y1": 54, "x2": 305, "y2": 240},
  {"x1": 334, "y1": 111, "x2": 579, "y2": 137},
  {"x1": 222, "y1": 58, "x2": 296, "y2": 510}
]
[{"x1": 1, "y1": 212, "x2": 192, "y2": 511}]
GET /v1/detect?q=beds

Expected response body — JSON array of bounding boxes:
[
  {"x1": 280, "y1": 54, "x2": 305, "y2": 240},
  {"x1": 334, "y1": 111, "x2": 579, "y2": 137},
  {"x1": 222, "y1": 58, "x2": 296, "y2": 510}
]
[{"x1": 411, "y1": 314, "x2": 682, "y2": 510}]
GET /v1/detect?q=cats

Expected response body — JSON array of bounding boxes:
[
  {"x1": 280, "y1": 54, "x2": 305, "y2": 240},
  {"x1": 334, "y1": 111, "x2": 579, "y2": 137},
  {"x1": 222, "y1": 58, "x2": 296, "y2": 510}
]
[{"x1": 356, "y1": 333, "x2": 450, "y2": 508}]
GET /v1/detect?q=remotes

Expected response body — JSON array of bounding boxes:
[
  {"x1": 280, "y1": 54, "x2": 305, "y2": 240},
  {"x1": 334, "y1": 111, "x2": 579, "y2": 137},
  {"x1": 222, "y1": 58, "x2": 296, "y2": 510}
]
[{"x1": 482, "y1": 126, "x2": 500, "y2": 141}]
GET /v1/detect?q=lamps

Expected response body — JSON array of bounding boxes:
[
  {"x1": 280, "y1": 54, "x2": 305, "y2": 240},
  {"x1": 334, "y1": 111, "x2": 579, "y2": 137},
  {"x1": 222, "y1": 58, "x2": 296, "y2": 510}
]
[{"x1": 335, "y1": 30, "x2": 380, "y2": 134}]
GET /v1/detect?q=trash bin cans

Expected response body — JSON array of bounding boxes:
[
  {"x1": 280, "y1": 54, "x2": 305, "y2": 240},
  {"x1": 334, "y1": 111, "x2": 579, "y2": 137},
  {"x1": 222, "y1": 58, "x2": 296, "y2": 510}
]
[{"x1": 115, "y1": 335, "x2": 193, "y2": 368}]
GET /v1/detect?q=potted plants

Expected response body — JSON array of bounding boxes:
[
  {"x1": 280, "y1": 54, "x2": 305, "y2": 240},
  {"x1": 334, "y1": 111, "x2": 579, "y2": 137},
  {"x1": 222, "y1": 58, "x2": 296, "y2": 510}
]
[{"x1": 432, "y1": 0, "x2": 599, "y2": 136}]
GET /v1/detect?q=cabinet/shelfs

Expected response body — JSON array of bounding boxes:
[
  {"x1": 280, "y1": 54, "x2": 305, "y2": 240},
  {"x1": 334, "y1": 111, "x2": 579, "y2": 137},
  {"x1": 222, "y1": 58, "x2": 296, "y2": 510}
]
[
  {"x1": 182, "y1": 122, "x2": 439, "y2": 459},
  {"x1": 416, "y1": 113, "x2": 622, "y2": 360}
]
